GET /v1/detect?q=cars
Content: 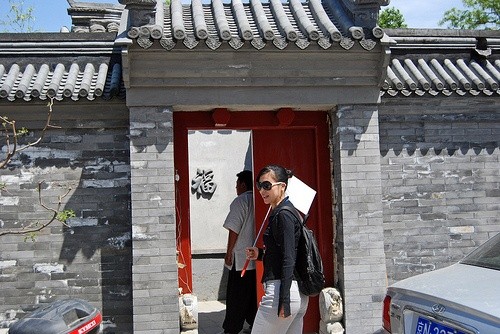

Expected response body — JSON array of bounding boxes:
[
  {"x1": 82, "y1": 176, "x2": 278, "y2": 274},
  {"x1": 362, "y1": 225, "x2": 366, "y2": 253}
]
[{"x1": 372, "y1": 227, "x2": 500, "y2": 334}]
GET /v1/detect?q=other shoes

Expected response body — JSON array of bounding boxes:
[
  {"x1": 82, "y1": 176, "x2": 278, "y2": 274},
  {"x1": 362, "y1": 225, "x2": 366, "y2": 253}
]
[{"x1": 243, "y1": 329, "x2": 252, "y2": 334}]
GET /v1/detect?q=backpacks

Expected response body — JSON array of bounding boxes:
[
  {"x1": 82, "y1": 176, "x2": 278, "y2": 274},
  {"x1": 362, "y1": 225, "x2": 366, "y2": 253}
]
[{"x1": 270, "y1": 207, "x2": 326, "y2": 298}]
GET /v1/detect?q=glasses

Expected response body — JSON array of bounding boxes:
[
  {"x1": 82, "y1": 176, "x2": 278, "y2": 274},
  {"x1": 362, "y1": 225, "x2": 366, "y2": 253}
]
[{"x1": 256, "y1": 181, "x2": 287, "y2": 191}]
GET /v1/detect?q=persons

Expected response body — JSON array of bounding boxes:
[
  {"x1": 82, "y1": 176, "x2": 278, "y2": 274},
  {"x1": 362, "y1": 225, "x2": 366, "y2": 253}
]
[
  {"x1": 219, "y1": 170, "x2": 258, "y2": 333},
  {"x1": 245, "y1": 165, "x2": 325, "y2": 333}
]
